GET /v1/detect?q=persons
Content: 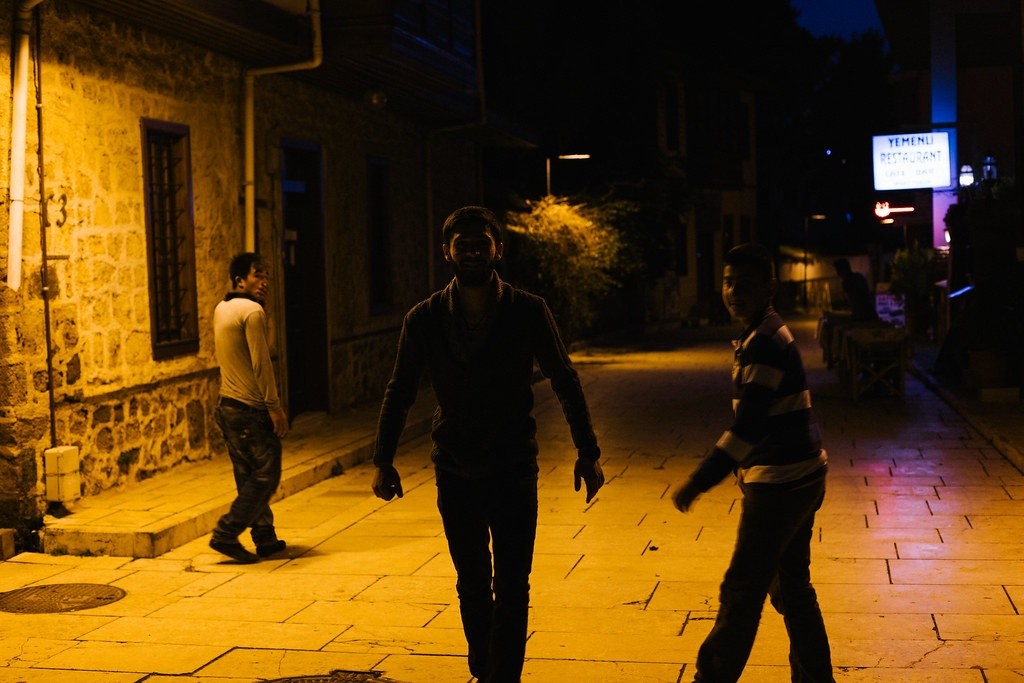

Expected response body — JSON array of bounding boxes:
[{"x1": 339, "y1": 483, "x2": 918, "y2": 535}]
[
  {"x1": 833, "y1": 257, "x2": 876, "y2": 323},
  {"x1": 373, "y1": 206, "x2": 606, "y2": 683},
  {"x1": 207, "y1": 253, "x2": 289, "y2": 563},
  {"x1": 674, "y1": 242, "x2": 838, "y2": 683}
]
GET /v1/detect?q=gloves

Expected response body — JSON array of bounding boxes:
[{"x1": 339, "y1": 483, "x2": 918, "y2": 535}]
[
  {"x1": 372, "y1": 466, "x2": 404, "y2": 501},
  {"x1": 574, "y1": 458, "x2": 607, "y2": 504}
]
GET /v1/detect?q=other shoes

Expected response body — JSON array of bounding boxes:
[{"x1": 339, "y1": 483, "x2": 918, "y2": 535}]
[
  {"x1": 209, "y1": 539, "x2": 258, "y2": 562},
  {"x1": 256, "y1": 540, "x2": 286, "y2": 558}
]
